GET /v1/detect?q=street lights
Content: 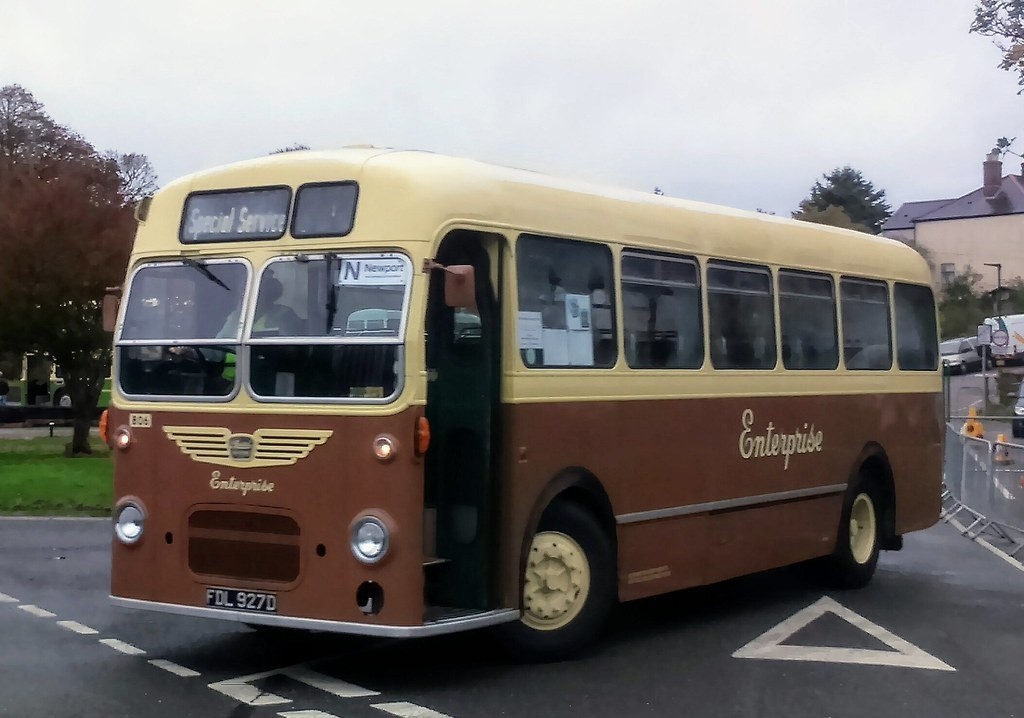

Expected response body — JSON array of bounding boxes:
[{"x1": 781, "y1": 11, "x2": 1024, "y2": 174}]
[{"x1": 984, "y1": 262, "x2": 1001, "y2": 315}]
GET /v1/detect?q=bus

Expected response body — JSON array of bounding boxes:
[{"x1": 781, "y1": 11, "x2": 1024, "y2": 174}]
[{"x1": 101, "y1": 146, "x2": 947, "y2": 658}]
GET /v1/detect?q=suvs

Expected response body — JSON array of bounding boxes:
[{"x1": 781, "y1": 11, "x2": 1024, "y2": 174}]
[{"x1": 940, "y1": 335, "x2": 991, "y2": 373}]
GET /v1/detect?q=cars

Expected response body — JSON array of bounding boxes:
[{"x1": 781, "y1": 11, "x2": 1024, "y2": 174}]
[{"x1": 1006, "y1": 376, "x2": 1024, "y2": 437}]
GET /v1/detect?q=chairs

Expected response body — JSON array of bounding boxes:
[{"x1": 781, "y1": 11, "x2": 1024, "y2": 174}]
[{"x1": 597, "y1": 328, "x2": 874, "y2": 370}]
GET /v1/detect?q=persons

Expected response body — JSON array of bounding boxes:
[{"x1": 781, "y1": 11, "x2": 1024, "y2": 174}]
[
  {"x1": 0, "y1": 372, "x2": 9, "y2": 406},
  {"x1": 170, "y1": 269, "x2": 296, "y2": 381}
]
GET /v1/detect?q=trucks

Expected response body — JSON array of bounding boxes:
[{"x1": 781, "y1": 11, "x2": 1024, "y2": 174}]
[{"x1": 984, "y1": 314, "x2": 1024, "y2": 366}]
